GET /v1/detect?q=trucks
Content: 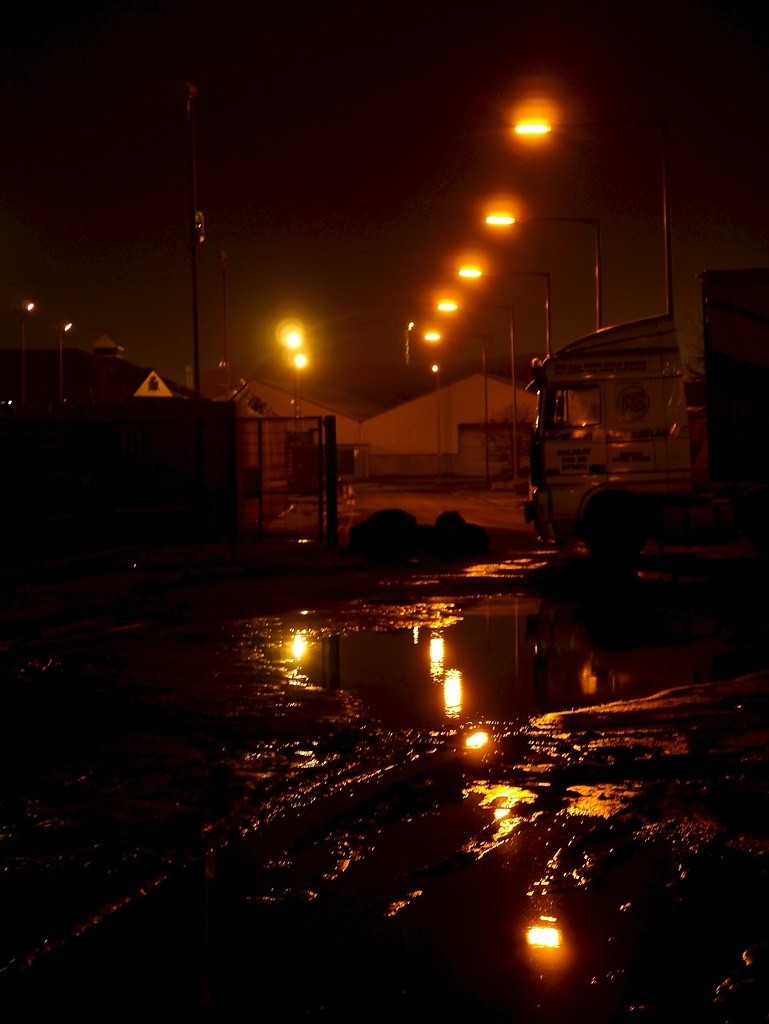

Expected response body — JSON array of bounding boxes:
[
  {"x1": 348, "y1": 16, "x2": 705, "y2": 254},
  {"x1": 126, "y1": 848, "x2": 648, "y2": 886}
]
[{"x1": 520, "y1": 266, "x2": 769, "y2": 557}]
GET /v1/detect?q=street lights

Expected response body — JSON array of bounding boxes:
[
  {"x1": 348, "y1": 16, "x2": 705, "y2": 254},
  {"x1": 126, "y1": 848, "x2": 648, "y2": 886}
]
[
  {"x1": 51, "y1": 321, "x2": 76, "y2": 404},
  {"x1": 511, "y1": 113, "x2": 675, "y2": 317},
  {"x1": 13, "y1": 300, "x2": 38, "y2": 403},
  {"x1": 427, "y1": 330, "x2": 491, "y2": 488},
  {"x1": 437, "y1": 297, "x2": 519, "y2": 480},
  {"x1": 177, "y1": 79, "x2": 203, "y2": 400},
  {"x1": 485, "y1": 209, "x2": 604, "y2": 332},
  {"x1": 458, "y1": 263, "x2": 553, "y2": 363},
  {"x1": 279, "y1": 323, "x2": 307, "y2": 445}
]
